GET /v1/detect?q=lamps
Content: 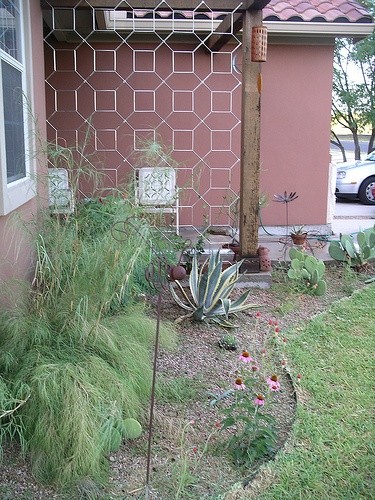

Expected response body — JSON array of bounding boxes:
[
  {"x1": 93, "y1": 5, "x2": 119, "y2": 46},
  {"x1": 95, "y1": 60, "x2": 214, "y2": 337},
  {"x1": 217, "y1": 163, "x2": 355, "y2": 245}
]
[{"x1": 251, "y1": 24, "x2": 270, "y2": 62}]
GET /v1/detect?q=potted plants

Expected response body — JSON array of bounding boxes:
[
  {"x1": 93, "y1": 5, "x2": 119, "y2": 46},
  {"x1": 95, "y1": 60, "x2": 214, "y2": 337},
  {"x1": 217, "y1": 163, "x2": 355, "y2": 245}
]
[{"x1": 289, "y1": 224, "x2": 307, "y2": 244}]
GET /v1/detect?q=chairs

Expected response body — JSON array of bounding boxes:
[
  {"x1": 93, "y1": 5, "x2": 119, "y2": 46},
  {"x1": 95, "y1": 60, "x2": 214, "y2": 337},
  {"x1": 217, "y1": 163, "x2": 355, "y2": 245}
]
[
  {"x1": 133, "y1": 166, "x2": 181, "y2": 237},
  {"x1": 47, "y1": 168, "x2": 76, "y2": 229}
]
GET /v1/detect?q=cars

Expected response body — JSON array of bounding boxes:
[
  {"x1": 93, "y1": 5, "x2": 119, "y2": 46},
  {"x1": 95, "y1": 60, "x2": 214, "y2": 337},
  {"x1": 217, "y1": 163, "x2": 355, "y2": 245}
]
[{"x1": 335, "y1": 150, "x2": 375, "y2": 205}]
